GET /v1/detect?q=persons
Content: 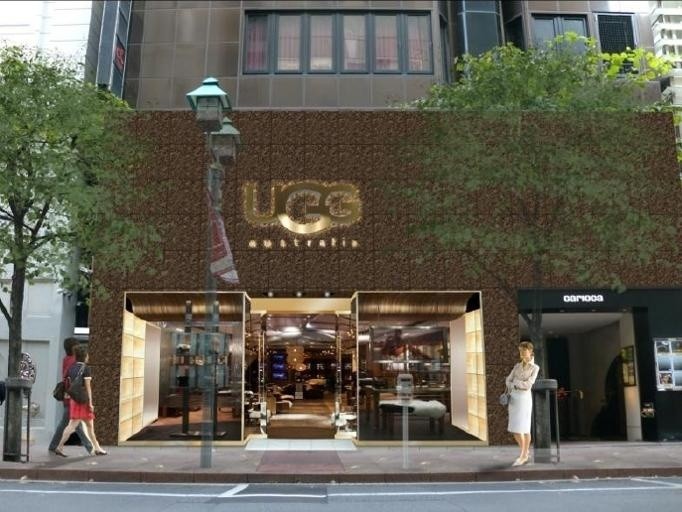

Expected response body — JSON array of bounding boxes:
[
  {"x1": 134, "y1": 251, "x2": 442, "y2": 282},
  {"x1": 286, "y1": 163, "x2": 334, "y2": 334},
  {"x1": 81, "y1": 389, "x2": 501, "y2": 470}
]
[
  {"x1": 55, "y1": 345, "x2": 109, "y2": 457},
  {"x1": 505, "y1": 341, "x2": 540, "y2": 468},
  {"x1": 48, "y1": 338, "x2": 93, "y2": 454}
]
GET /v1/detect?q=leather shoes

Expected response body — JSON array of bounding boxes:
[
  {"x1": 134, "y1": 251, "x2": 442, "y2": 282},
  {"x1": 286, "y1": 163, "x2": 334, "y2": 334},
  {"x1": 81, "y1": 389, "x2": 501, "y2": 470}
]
[
  {"x1": 89, "y1": 449, "x2": 107, "y2": 455},
  {"x1": 48, "y1": 447, "x2": 66, "y2": 457},
  {"x1": 513, "y1": 456, "x2": 528, "y2": 466}
]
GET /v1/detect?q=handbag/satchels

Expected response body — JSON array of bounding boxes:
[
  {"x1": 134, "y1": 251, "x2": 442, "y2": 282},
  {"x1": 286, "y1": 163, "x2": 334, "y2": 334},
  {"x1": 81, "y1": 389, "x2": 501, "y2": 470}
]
[
  {"x1": 54, "y1": 381, "x2": 65, "y2": 400},
  {"x1": 67, "y1": 377, "x2": 89, "y2": 404},
  {"x1": 499, "y1": 392, "x2": 510, "y2": 404}
]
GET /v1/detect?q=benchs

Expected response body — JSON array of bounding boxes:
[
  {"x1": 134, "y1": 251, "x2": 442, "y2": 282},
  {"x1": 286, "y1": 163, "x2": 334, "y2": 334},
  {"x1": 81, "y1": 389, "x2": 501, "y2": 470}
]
[{"x1": 268, "y1": 413, "x2": 338, "y2": 438}]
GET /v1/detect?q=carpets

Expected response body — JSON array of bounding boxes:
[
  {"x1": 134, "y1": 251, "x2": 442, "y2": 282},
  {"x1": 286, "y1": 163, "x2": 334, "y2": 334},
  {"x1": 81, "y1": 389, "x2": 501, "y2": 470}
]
[{"x1": 242, "y1": 437, "x2": 358, "y2": 451}]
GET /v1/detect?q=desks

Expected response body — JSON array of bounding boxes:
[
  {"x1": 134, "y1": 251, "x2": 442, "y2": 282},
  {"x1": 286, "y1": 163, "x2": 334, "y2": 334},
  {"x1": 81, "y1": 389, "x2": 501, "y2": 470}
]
[{"x1": 358, "y1": 376, "x2": 451, "y2": 434}]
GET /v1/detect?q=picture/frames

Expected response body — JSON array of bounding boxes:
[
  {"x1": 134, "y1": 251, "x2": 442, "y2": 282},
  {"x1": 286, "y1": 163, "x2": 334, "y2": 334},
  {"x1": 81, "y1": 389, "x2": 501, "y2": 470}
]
[{"x1": 619, "y1": 345, "x2": 637, "y2": 386}]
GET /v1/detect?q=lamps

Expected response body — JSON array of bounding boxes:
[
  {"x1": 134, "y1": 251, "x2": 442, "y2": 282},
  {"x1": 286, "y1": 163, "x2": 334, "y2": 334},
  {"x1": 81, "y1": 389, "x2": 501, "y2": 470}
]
[{"x1": 640, "y1": 398, "x2": 656, "y2": 419}]
